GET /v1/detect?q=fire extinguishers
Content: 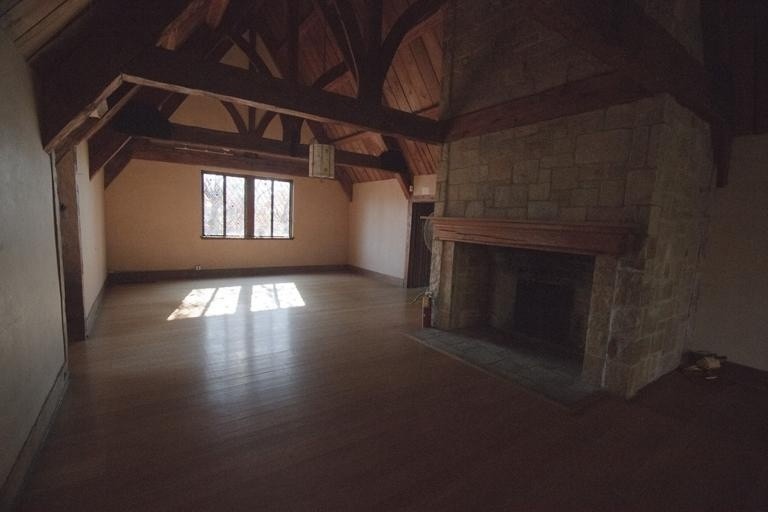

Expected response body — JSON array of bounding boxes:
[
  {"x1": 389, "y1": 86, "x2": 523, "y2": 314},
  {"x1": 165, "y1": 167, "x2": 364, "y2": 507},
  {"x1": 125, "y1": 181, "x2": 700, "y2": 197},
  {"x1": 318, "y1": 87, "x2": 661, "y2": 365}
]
[{"x1": 409, "y1": 288, "x2": 437, "y2": 328}]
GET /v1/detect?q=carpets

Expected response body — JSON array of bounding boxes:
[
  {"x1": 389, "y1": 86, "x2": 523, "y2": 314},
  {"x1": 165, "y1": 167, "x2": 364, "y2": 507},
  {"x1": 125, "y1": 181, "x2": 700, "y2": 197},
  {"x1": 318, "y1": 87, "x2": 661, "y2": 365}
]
[{"x1": 400, "y1": 323, "x2": 608, "y2": 414}]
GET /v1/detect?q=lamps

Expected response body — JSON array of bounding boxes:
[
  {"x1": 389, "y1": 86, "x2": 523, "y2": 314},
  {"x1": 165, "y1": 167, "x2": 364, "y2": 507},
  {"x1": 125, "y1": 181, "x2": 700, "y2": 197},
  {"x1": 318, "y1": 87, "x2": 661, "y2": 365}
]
[{"x1": 308, "y1": 123, "x2": 335, "y2": 183}]
